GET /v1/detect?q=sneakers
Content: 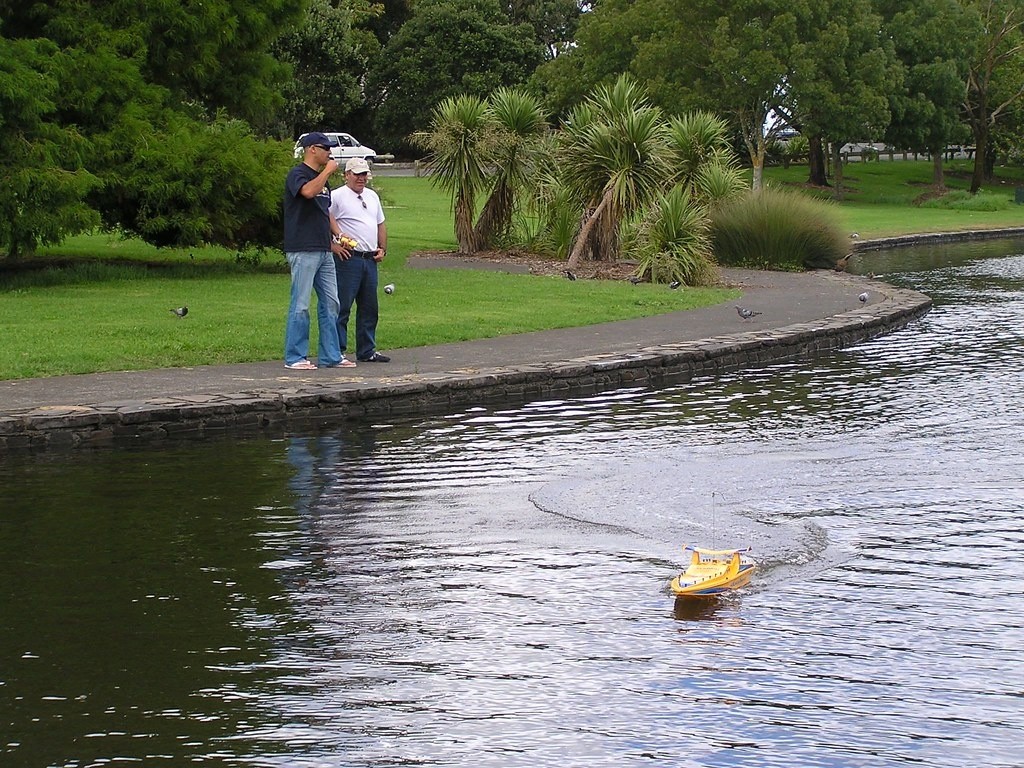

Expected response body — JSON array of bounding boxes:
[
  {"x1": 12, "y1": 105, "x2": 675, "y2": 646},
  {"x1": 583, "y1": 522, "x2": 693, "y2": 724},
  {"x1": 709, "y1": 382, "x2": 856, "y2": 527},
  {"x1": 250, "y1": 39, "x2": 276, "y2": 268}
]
[
  {"x1": 356, "y1": 352, "x2": 390, "y2": 362},
  {"x1": 339, "y1": 354, "x2": 349, "y2": 360}
]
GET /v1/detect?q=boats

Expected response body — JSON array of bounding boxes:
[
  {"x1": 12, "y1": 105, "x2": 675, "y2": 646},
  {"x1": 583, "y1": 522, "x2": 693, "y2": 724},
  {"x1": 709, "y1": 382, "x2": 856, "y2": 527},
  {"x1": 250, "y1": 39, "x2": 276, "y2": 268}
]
[{"x1": 669, "y1": 488, "x2": 762, "y2": 596}]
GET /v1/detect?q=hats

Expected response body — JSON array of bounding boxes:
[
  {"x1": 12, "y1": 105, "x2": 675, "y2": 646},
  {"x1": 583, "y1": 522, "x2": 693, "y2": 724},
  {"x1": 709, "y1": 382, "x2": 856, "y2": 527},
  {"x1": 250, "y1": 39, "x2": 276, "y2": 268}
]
[
  {"x1": 345, "y1": 158, "x2": 371, "y2": 174},
  {"x1": 301, "y1": 132, "x2": 339, "y2": 147}
]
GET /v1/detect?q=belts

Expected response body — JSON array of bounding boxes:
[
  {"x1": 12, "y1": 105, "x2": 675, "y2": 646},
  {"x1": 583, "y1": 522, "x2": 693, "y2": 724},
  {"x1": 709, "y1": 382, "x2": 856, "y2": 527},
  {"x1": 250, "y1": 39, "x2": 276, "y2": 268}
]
[{"x1": 345, "y1": 248, "x2": 379, "y2": 259}]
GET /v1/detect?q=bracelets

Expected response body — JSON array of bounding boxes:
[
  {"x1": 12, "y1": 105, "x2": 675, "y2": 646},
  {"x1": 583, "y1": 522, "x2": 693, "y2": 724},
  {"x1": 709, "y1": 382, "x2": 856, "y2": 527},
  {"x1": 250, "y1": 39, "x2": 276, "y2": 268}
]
[
  {"x1": 378, "y1": 247, "x2": 387, "y2": 256},
  {"x1": 339, "y1": 233, "x2": 343, "y2": 239}
]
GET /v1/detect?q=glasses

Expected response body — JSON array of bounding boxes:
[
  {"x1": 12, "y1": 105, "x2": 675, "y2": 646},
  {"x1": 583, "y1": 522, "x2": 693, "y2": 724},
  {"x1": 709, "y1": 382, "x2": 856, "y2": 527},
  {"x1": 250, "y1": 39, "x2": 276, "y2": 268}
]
[
  {"x1": 358, "y1": 195, "x2": 367, "y2": 209},
  {"x1": 309, "y1": 145, "x2": 330, "y2": 151}
]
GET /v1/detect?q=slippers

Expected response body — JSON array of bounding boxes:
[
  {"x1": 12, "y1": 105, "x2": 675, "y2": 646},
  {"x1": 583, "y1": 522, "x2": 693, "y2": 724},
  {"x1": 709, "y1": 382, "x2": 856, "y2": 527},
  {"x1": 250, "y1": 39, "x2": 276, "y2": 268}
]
[
  {"x1": 284, "y1": 359, "x2": 318, "y2": 369},
  {"x1": 329, "y1": 360, "x2": 357, "y2": 367}
]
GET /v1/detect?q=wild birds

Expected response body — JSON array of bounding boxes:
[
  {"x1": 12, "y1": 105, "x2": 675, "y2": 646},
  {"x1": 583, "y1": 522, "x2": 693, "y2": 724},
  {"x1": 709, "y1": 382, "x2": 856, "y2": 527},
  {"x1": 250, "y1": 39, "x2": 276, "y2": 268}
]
[
  {"x1": 629, "y1": 275, "x2": 641, "y2": 285},
  {"x1": 167, "y1": 304, "x2": 190, "y2": 318},
  {"x1": 384, "y1": 282, "x2": 394, "y2": 295},
  {"x1": 669, "y1": 281, "x2": 682, "y2": 290},
  {"x1": 733, "y1": 305, "x2": 763, "y2": 323},
  {"x1": 566, "y1": 272, "x2": 576, "y2": 281},
  {"x1": 858, "y1": 292, "x2": 870, "y2": 304},
  {"x1": 848, "y1": 231, "x2": 861, "y2": 240}
]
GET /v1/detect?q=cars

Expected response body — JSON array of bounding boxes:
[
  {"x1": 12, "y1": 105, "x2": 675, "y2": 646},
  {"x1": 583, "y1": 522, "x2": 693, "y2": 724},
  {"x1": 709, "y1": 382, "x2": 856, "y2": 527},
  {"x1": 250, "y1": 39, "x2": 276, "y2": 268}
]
[
  {"x1": 293, "y1": 131, "x2": 376, "y2": 165},
  {"x1": 777, "y1": 125, "x2": 801, "y2": 141}
]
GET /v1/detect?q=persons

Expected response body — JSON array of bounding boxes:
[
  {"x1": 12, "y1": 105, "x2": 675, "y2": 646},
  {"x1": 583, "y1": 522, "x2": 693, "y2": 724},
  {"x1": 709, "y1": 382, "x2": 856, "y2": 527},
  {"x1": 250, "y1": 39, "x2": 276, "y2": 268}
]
[
  {"x1": 329, "y1": 158, "x2": 391, "y2": 361},
  {"x1": 283, "y1": 132, "x2": 357, "y2": 370}
]
